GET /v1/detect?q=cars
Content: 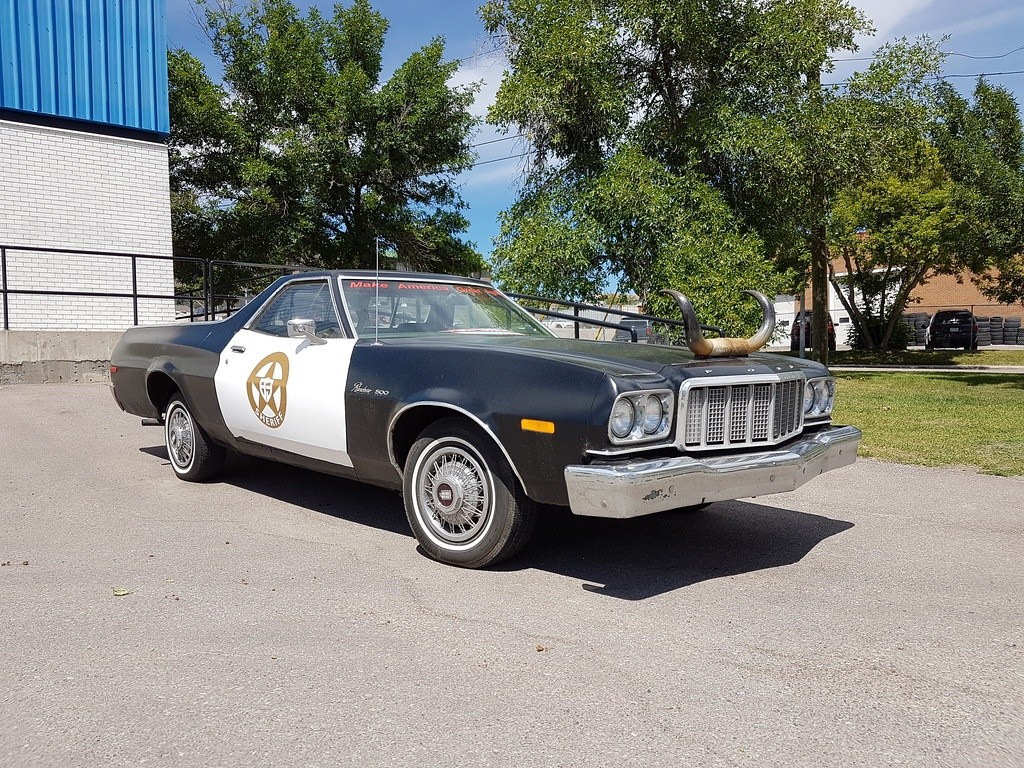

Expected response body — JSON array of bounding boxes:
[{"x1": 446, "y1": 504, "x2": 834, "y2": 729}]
[
  {"x1": 109, "y1": 270, "x2": 862, "y2": 569},
  {"x1": 615, "y1": 315, "x2": 656, "y2": 342},
  {"x1": 791, "y1": 310, "x2": 839, "y2": 352},
  {"x1": 921, "y1": 309, "x2": 979, "y2": 350}
]
